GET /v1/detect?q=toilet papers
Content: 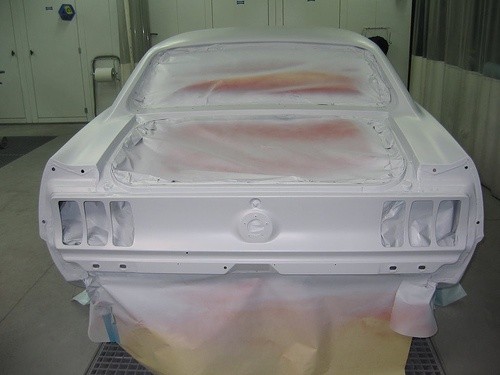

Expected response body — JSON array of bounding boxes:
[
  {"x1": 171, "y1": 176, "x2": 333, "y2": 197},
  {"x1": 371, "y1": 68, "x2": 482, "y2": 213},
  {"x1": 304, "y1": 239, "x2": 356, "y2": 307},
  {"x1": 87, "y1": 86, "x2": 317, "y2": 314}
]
[{"x1": 95, "y1": 67, "x2": 114, "y2": 82}]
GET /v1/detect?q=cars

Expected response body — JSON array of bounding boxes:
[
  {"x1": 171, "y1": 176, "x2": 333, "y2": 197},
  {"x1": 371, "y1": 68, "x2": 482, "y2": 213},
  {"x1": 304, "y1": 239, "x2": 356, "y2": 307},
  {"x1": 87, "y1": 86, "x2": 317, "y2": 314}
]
[{"x1": 37, "y1": 30, "x2": 485, "y2": 286}]
